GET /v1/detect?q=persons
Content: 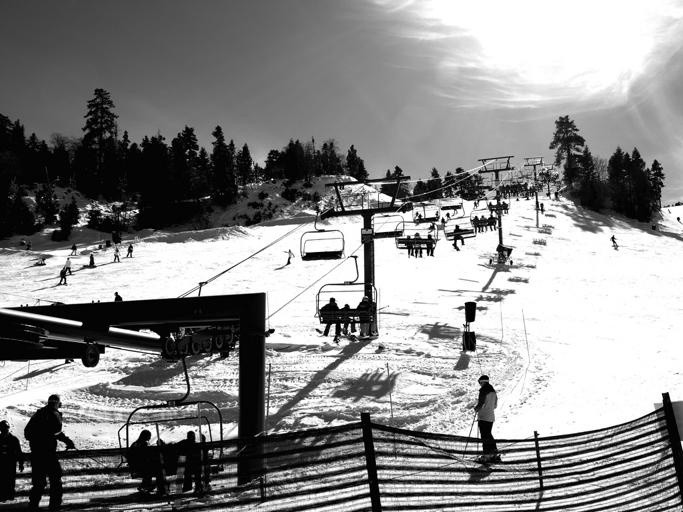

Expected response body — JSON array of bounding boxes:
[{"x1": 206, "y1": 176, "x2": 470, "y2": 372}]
[
  {"x1": 113, "y1": 247, "x2": 119, "y2": 262},
  {"x1": 41, "y1": 256, "x2": 45, "y2": 264},
  {"x1": 22, "y1": 393, "x2": 74, "y2": 511},
  {"x1": 126, "y1": 244, "x2": 133, "y2": 257},
  {"x1": 89, "y1": 254, "x2": 94, "y2": 265},
  {"x1": 177, "y1": 431, "x2": 206, "y2": 493},
  {"x1": 26, "y1": 239, "x2": 32, "y2": 250},
  {"x1": 59, "y1": 267, "x2": 67, "y2": 285},
  {"x1": 405, "y1": 180, "x2": 531, "y2": 259},
  {"x1": 609, "y1": 235, "x2": 618, "y2": 247},
  {"x1": 319, "y1": 298, "x2": 342, "y2": 336},
  {"x1": 356, "y1": 296, "x2": 374, "y2": 337},
  {"x1": 283, "y1": 249, "x2": 295, "y2": 266},
  {"x1": 114, "y1": 291, "x2": 122, "y2": 301},
  {"x1": 64, "y1": 258, "x2": 71, "y2": 275},
  {"x1": 338, "y1": 304, "x2": 357, "y2": 334},
  {"x1": 0, "y1": 419, "x2": 25, "y2": 502},
  {"x1": 70, "y1": 243, "x2": 76, "y2": 256},
  {"x1": 473, "y1": 374, "x2": 500, "y2": 462},
  {"x1": 64, "y1": 358, "x2": 73, "y2": 364},
  {"x1": 129, "y1": 430, "x2": 157, "y2": 492}
]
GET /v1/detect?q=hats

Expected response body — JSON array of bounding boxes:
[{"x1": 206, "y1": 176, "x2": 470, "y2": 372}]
[{"x1": 478, "y1": 375, "x2": 490, "y2": 383}]
